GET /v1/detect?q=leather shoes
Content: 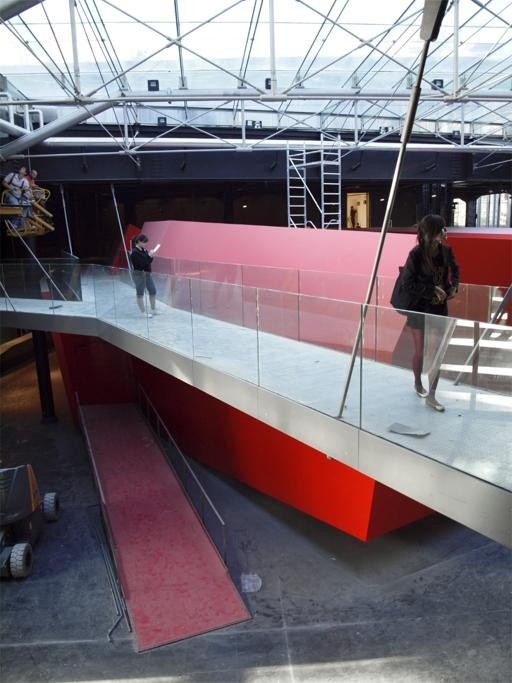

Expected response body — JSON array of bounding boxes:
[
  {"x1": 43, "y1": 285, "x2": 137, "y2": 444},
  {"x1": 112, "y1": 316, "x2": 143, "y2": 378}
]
[
  {"x1": 411, "y1": 384, "x2": 429, "y2": 398},
  {"x1": 425, "y1": 397, "x2": 445, "y2": 411}
]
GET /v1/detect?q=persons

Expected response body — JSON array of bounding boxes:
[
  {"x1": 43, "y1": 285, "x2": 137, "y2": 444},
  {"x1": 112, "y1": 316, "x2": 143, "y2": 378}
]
[
  {"x1": 21, "y1": 168, "x2": 43, "y2": 228},
  {"x1": 131, "y1": 234, "x2": 164, "y2": 318},
  {"x1": 2, "y1": 164, "x2": 29, "y2": 229},
  {"x1": 405, "y1": 213, "x2": 461, "y2": 413}
]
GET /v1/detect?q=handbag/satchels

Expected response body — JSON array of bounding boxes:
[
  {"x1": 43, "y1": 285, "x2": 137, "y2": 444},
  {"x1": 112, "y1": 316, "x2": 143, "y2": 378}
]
[{"x1": 389, "y1": 265, "x2": 426, "y2": 316}]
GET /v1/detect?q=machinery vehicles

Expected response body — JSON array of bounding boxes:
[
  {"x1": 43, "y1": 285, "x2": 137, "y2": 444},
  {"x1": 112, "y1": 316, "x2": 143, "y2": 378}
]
[{"x1": 2, "y1": 464, "x2": 61, "y2": 577}]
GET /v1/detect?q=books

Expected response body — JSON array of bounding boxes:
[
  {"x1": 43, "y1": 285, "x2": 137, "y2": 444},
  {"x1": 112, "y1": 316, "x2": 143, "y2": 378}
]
[{"x1": 153, "y1": 243, "x2": 160, "y2": 253}]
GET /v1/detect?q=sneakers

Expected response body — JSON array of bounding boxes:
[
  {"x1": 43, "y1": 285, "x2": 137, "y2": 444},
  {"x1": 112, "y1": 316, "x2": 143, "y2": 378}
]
[{"x1": 143, "y1": 309, "x2": 162, "y2": 318}]
